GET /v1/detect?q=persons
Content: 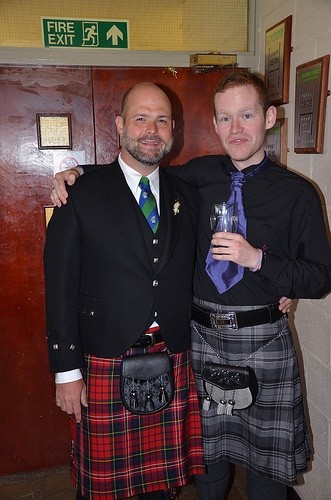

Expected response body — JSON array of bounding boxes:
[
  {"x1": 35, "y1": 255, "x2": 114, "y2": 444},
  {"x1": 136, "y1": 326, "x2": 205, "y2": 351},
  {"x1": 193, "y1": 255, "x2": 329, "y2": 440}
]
[
  {"x1": 42, "y1": 81, "x2": 292, "y2": 500},
  {"x1": 50, "y1": 71, "x2": 331, "y2": 500}
]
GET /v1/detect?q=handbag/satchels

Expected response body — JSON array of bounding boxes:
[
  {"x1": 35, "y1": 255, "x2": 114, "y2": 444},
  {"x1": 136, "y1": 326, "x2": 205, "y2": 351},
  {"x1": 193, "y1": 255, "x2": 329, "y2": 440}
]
[
  {"x1": 201, "y1": 361, "x2": 258, "y2": 417},
  {"x1": 120, "y1": 352, "x2": 175, "y2": 416}
]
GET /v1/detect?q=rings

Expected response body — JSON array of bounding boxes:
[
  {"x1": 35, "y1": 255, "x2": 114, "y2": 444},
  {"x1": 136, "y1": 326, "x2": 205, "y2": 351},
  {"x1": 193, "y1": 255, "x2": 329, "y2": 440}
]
[{"x1": 50, "y1": 186, "x2": 56, "y2": 191}]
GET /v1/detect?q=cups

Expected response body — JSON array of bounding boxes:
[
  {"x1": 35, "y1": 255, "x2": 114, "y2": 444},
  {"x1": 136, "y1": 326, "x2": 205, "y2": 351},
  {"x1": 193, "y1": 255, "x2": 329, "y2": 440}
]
[{"x1": 213, "y1": 202, "x2": 234, "y2": 238}]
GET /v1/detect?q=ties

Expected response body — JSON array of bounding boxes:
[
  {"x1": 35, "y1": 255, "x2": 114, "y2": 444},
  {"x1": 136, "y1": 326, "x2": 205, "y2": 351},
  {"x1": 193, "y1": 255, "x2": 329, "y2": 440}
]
[
  {"x1": 138, "y1": 176, "x2": 160, "y2": 234},
  {"x1": 205, "y1": 155, "x2": 269, "y2": 295}
]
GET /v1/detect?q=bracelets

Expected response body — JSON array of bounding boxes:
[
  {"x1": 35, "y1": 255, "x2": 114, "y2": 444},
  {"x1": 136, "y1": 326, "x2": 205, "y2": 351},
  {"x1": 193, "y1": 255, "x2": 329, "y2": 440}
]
[{"x1": 250, "y1": 248, "x2": 263, "y2": 272}]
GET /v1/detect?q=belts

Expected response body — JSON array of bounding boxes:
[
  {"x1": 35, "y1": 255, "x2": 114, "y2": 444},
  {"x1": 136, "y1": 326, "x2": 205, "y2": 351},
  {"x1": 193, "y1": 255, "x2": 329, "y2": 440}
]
[
  {"x1": 131, "y1": 330, "x2": 163, "y2": 349},
  {"x1": 191, "y1": 301, "x2": 286, "y2": 332}
]
